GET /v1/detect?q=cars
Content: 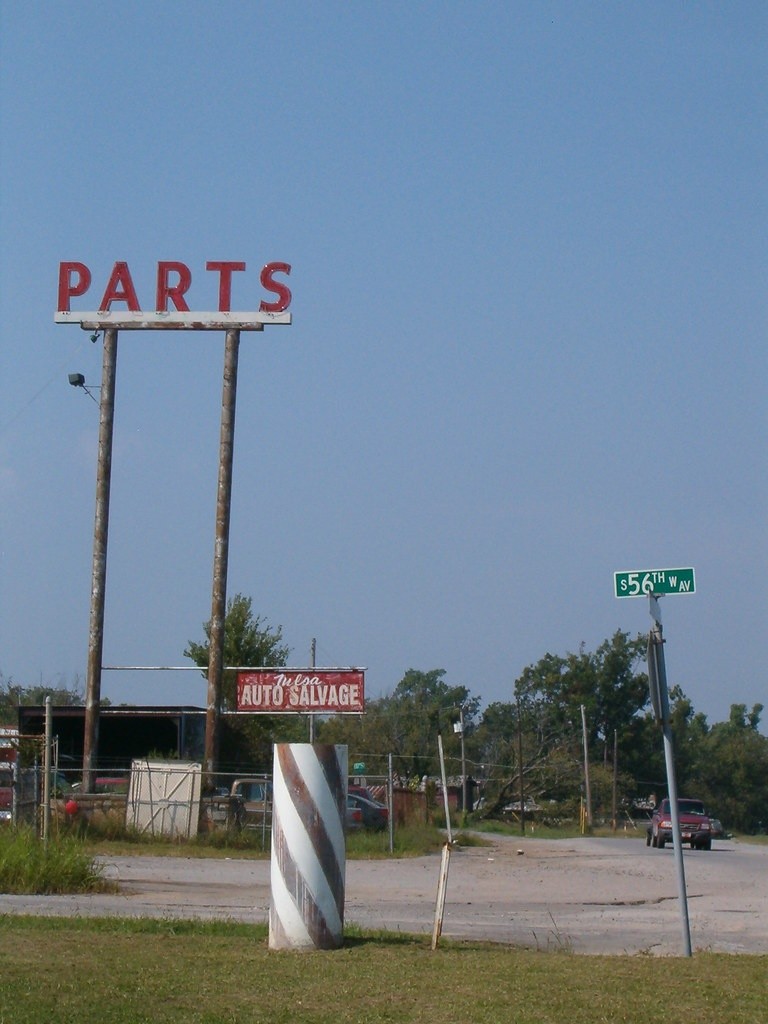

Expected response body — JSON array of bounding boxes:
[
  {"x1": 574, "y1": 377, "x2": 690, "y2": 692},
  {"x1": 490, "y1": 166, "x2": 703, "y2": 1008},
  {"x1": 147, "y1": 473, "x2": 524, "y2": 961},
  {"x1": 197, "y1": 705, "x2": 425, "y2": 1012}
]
[
  {"x1": 348, "y1": 785, "x2": 388, "y2": 807},
  {"x1": 213, "y1": 787, "x2": 229, "y2": 798},
  {"x1": 709, "y1": 818, "x2": 724, "y2": 839},
  {"x1": 346, "y1": 793, "x2": 389, "y2": 833},
  {"x1": 72, "y1": 776, "x2": 130, "y2": 793},
  {"x1": 472, "y1": 793, "x2": 545, "y2": 822}
]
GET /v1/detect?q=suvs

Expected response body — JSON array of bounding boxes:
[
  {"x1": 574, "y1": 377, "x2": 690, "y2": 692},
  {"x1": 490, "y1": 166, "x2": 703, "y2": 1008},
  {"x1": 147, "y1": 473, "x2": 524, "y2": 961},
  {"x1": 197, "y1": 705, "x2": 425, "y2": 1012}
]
[{"x1": 645, "y1": 797, "x2": 713, "y2": 850}]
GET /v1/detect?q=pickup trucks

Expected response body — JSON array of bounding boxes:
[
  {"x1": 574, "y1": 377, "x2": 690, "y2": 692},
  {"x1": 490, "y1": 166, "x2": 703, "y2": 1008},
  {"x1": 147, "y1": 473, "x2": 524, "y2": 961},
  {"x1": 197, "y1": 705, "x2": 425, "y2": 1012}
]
[{"x1": 198, "y1": 779, "x2": 273, "y2": 834}]
